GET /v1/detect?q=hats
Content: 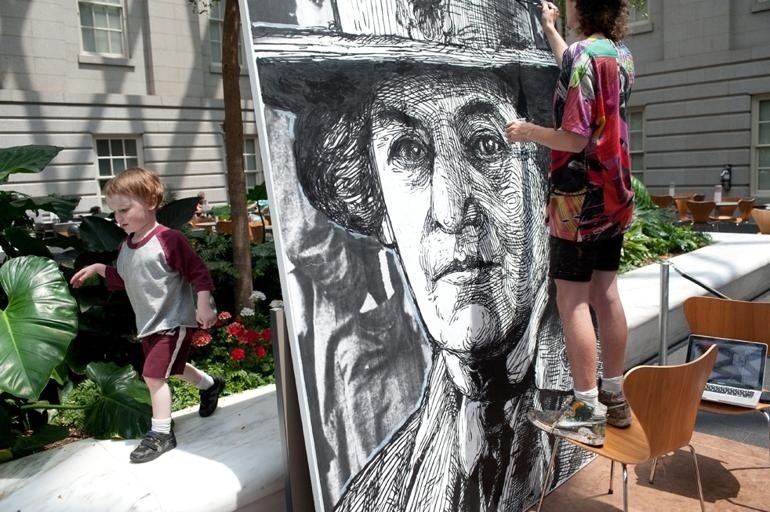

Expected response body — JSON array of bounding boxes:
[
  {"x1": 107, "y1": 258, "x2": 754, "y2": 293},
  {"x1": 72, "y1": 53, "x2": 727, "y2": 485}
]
[{"x1": 252, "y1": 0, "x2": 561, "y2": 116}]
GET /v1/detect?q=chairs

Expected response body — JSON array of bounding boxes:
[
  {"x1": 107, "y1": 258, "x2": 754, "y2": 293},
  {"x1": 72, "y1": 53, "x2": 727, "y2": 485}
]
[
  {"x1": 528, "y1": 342, "x2": 720, "y2": 512},
  {"x1": 650, "y1": 192, "x2": 770, "y2": 235},
  {"x1": 683, "y1": 297, "x2": 770, "y2": 424},
  {"x1": 189, "y1": 212, "x2": 270, "y2": 241}
]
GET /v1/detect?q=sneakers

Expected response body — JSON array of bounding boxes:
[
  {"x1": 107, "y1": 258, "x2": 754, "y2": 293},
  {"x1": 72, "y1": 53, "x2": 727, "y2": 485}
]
[
  {"x1": 596, "y1": 389, "x2": 633, "y2": 430},
  {"x1": 129, "y1": 429, "x2": 177, "y2": 463},
  {"x1": 198, "y1": 377, "x2": 226, "y2": 418},
  {"x1": 527, "y1": 399, "x2": 607, "y2": 446}
]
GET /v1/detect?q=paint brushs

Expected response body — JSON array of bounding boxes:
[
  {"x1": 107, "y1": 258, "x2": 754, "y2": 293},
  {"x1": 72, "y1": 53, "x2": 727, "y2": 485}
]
[{"x1": 522, "y1": 0, "x2": 552, "y2": 10}]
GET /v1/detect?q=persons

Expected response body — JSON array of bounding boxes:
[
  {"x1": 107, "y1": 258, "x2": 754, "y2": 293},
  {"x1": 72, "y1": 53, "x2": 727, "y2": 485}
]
[
  {"x1": 250, "y1": 0, "x2": 614, "y2": 512},
  {"x1": 70, "y1": 168, "x2": 225, "y2": 464},
  {"x1": 500, "y1": 1, "x2": 635, "y2": 448}
]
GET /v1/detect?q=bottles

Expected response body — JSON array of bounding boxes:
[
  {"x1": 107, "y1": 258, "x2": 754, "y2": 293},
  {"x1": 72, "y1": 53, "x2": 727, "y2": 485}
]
[
  {"x1": 668, "y1": 181, "x2": 675, "y2": 197},
  {"x1": 713, "y1": 185, "x2": 722, "y2": 203}
]
